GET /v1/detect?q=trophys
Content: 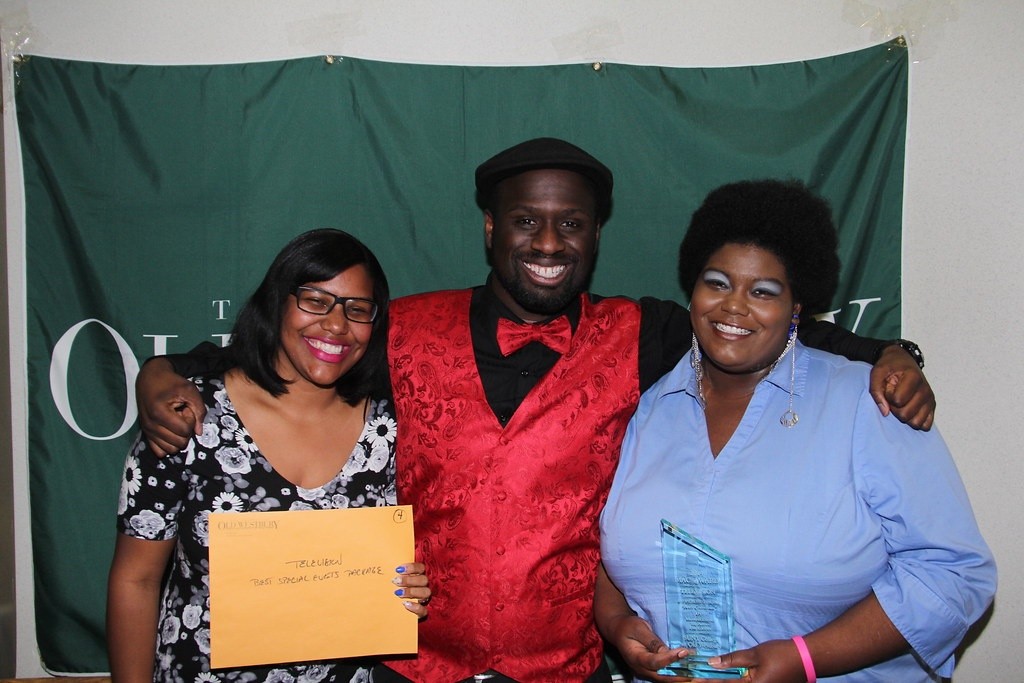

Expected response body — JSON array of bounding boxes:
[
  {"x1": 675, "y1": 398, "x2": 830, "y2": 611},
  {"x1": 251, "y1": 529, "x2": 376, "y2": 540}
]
[{"x1": 655, "y1": 517, "x2": 746, "y2": 681}]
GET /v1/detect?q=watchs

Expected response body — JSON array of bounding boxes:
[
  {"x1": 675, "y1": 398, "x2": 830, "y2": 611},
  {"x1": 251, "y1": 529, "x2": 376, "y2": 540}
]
[{"x1": 873, "y1": 338, "x2": 925, "y2": 369}]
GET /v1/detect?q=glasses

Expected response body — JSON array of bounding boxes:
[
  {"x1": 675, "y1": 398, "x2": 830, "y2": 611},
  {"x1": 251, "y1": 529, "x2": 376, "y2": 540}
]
[{"x1": 289, "y1": 284, "x2": 384, "y2": 324}]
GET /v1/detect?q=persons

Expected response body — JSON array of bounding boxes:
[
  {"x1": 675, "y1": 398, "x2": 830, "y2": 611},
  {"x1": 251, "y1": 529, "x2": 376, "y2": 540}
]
[
  {"x1": 593, "y1": 173, "x2": 1000, "y2": 683},
  {"x1": 104, "y1": 229, "x2": 432, "y2": 683},
  {"x1": 135, "y1": 136, "x2": 937, "y2": 683}
]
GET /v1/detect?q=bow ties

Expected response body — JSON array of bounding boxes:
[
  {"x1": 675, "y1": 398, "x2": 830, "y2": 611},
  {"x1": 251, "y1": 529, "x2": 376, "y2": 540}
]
[{"x1": 496, "y1": 313, "x2": 572, "y2": 357}]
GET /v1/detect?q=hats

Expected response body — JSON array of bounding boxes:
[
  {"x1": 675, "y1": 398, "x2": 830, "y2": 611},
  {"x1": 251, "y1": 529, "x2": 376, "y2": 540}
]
[{"x1": 474, "y1": 137, "x2": 613, "y2": 198}]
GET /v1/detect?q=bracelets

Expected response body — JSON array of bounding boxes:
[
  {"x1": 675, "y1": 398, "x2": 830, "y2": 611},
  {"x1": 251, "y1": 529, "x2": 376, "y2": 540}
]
[{"x1": 791, "y1": 636, "x2": 817, "y2": 683}]
